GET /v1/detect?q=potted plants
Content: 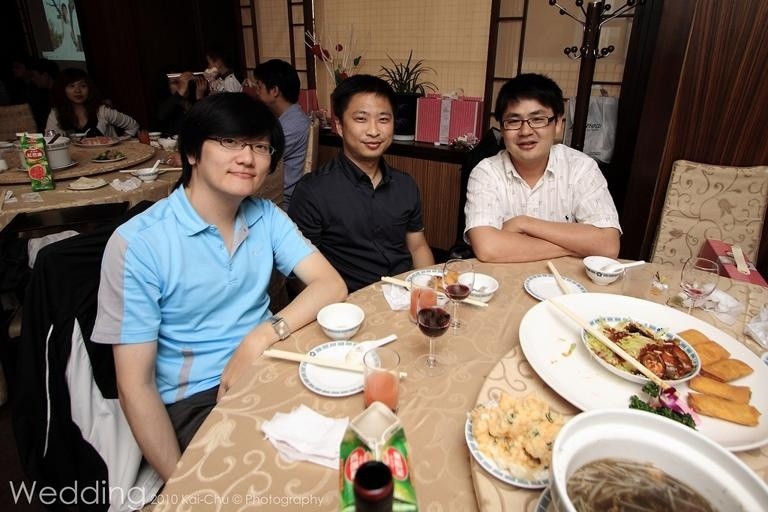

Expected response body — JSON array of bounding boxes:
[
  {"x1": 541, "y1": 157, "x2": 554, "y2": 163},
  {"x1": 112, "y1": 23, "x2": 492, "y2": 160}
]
[{"x1": 376, "y1": 50, "x2": 439, "y2": 141}]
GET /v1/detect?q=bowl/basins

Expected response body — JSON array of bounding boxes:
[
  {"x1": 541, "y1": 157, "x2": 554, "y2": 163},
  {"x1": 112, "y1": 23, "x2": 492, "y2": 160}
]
[
  {"x1": 583, "y1": 255, "x2": 625, "y2": 286},
  {"x1": 457, "y1": 272, "x2": 499, "y2": 303},
  {"x1": 17, "y1": 136, "x2": 71, "y2": 169},
  {"x1": 137, "y1": 168, "x2": 159, "y2": 182},
  {"x1": 148, "y1": 132, "x2": 162, "y2": 140},
  {"x1": 316, "y1": 302, "x2": 365, "y2": 340},
  {"x1": 550, "y1": 409, "x2": 768, "y2": 512}
]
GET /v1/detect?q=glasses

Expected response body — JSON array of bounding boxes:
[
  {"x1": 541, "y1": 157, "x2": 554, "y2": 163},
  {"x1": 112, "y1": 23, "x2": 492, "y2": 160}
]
[
  {"x1": 500, "y1": 115, "x2": 556, "y2": 131},
  {"x1": 206, "y1": 135, "x2": 277, "y2": 156}
]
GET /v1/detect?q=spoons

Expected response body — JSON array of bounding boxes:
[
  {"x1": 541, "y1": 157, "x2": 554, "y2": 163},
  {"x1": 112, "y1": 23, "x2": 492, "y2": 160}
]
[
  {"x1": 344, "y1": 333, "x2": 398, "y2": 363},
  {"x1": 600, "y1": 260, "x2": 646, "y2": 272}
]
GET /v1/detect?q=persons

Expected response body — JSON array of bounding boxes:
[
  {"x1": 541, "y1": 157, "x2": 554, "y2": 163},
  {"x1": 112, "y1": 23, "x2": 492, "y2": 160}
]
[
  {"x1": 0, "y1": 54, "x2": 58, "y2": 101},
  {"x1": 42, "y1": 4, "x2": 86, "y2": 61},
  {"x1": 253, "y1": 59, "x2": 312, "y2": 213},
  {"x1": 463, "y1": 74, "x2": 624, "y2": 262},
  {"x1": 289, "y1": 74, "x2": 435, "y2": 290},
  {"x1": 202, "y1": 45, "x2": 244, "y2": 94},
  {"x1": 90, "y1": 90, "x2": 348, "y2": 483},
  {"x1": 44, "y1": 68, "x2": 140, "y2": 138},
  {"x1": 151, "y1": 69, "x2": 207, "y2": 138}
]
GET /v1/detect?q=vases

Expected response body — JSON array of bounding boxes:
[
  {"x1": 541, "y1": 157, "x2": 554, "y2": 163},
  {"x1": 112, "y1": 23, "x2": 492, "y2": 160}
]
[{"x1": 330, "y1": 95, "x2": 338, "y2": 134}]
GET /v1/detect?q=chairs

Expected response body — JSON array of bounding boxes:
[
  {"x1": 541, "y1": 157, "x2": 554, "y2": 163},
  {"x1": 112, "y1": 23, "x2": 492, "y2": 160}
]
[
  {"x1": 650, "y1": 159, "x2": 768, "y2": 268},
  {"x1": 13, "y1": 200, "x2": 159, "y2": 510},
  {"x1": 303, "y1": 118, "x2": 319, "y2": 175},
  {"x1": 0, "y1": 104, "x2": 38, "y2": 141},
  {"x1": 251, "y1": 155, "x2": 289, "y2": 315}
]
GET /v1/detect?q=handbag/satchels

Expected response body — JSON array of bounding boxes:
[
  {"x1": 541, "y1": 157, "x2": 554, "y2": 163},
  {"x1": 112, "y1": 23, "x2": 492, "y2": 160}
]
[{"x1": 560, "y1": 89, "x2": 619, "y2": 167}]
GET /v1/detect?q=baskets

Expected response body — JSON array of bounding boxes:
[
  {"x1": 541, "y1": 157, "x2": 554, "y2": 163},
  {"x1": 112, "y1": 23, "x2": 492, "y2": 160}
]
[{"x1": 696, "y1": 237, "x2": 764, "y2": 291}]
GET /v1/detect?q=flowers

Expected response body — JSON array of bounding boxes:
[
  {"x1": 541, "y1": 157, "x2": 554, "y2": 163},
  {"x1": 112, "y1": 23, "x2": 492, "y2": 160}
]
[{"x1": 303, "y1": 24, "x2": 368, "y2": 89}]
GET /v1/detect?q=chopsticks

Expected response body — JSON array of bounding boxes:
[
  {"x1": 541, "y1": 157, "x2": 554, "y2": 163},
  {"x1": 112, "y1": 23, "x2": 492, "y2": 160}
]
[
  {"x1": 264, "y1": 349, "x2": 408, "y2": 379},
  {"x1": 381, "y1": 276, "x2": 489, "y2": 308},
  {"x1": 549, "y1": 297, "x2": 669, "y2": 390},
  {"x1": 546, "y1": 261, "x2": 569, "y2": 294},
  {"x1": 120, "y1": 167, "x2": 183, "y2": 173}
]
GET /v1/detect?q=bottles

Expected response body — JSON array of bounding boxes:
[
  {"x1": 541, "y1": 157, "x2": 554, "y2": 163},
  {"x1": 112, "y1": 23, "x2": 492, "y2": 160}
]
[
  {"x1": 337, "y1": 399, "x2": 419, "y2": 512},
  {"x1": 19, "y1": 135, "x2": 56, "y2": 191},
  {"x1": 351, "y1": 460, "x2": 394, "y2": 511}
]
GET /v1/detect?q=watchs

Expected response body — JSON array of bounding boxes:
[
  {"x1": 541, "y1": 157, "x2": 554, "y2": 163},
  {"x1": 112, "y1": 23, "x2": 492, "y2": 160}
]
[{"x1": 269, "y1": 314, "x2": 293, "y2": 342}]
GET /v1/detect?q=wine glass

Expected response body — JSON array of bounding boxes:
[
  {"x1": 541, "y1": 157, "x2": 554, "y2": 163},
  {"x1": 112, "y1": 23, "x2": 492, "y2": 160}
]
[
  {"x1": 414, "y1": 290, "x2": 452, "y2": 378},
  {"x1": 442, "y1": 259, "x2": 475, "y2": 335},
  {"x1": 680, "y1": 257, "x2": 720, "y2": 315}
]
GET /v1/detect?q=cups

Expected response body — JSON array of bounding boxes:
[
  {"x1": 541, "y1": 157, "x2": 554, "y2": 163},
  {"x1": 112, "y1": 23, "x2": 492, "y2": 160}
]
[
  {"x1": 408, "y1": 274, "x2": 438, "y2": 325},
  {"x1": 363, "y1": 347, "x2": 401, "y2": 417},
  {"x1": 623, "y1": 267, "x2": 653, "y2": 300}
]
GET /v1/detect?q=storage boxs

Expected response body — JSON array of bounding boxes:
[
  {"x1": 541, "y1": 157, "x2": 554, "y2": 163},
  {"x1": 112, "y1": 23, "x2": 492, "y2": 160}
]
[
  {"x1": 414, "y1": 94, "x2": 484, "y2": 145},
  {"x1": 697, "y1": 239, "x2": 767, "y2": 286}
]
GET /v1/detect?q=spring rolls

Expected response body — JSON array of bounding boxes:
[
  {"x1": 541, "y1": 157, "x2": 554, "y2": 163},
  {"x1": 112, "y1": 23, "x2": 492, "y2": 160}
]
[
  {"x1": 688, "y1": 375, "x2": 752, "y2": 406},
  {"x1": 677, "y1": 328, "x2": 712, "y2": 347},
  {"x1": 687, "y1": 392, "x2": 762, "y2": 427},
  {"x1": 700, "y1": 358, "x2": 753, "y2": 383},
  {"x1": 695, "y1": 342, "x2": 731, "y2": 373}
]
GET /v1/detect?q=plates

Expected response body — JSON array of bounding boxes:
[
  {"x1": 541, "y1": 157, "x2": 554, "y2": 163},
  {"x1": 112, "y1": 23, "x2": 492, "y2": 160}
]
[
  {"x1": 523, "y1": 272, "x2": 588, "y2": 303},
  {"x1": 17, "y1": 160, "x2": 79, "y2": 172},
  {"x1": 67, "y1": 178, "x2": 109, "y2": 190},
  {"x1": 90, "y1": 153, "x2": 127, "y2": 162},
  {"x1": 519, "y1": 292, "x2": 768, "y2": 453},
  {"x1": 71, "y1": 136, "x2": 120, "y2": 147},
  {"x1": 404, "y1": 268, "x2": 448, "y2": 292},
  {"x1": 299, "y1": 340, "x2": 381, "y2": 397},
  {"x1": 464, "y1": 398, "x2": 549, "y2": 489},
  {"x1": 581, "y1": 314, "x2": 701, "y2": 386}
]
